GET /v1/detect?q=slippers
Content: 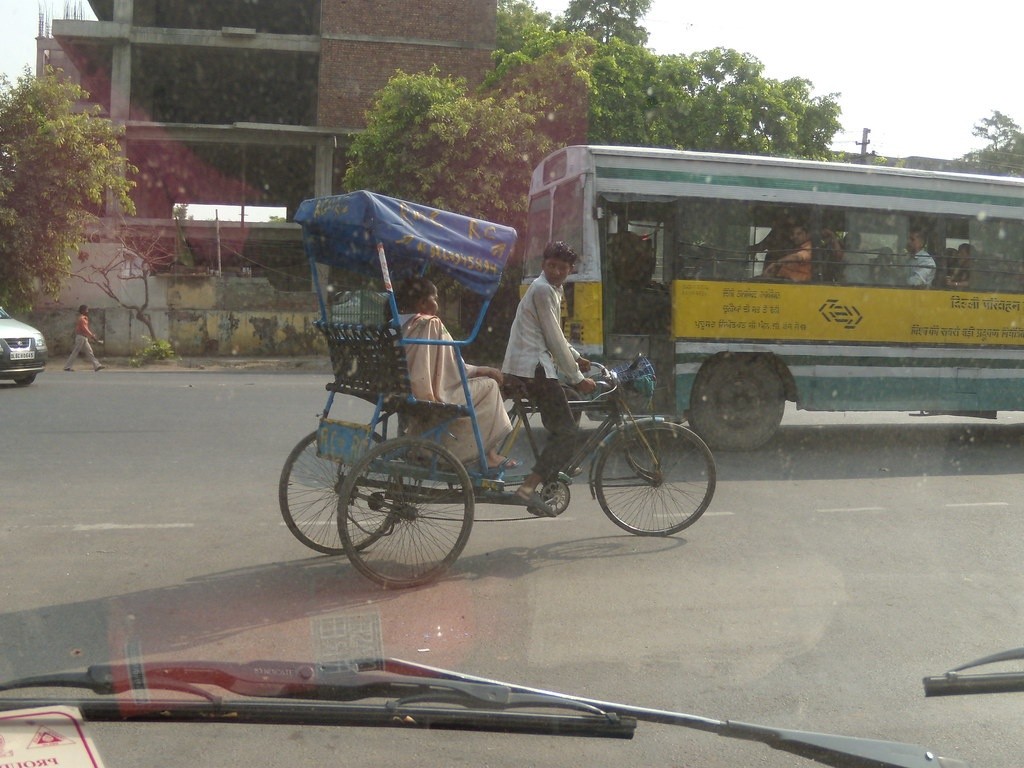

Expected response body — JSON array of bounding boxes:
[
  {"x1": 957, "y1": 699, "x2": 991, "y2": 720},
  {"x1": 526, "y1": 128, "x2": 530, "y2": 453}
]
[
  {"x1": 552, "y1": 465, "x2": 582, "y2": 482},
  {"x1": 486, "y1": 455, "x2": 523, "y2": 472},
  {"x1": 516, "y1": 488, "x2": 556, "y2": 517}
]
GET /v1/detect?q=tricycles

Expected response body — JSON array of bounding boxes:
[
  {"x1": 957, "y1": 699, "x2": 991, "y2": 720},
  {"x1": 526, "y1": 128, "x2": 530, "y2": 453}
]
[{"x1": 280, "y1": 186, "x2": 722, "y2": 591}]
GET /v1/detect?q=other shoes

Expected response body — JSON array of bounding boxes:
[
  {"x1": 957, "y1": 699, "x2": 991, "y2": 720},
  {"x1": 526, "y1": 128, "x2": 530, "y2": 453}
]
[
  {"x1": 64, "y1": 368, "x2": 74, "y2": 371},
  {"x1": 95, "y1": 366, "x2": 105, "y2": 371}
]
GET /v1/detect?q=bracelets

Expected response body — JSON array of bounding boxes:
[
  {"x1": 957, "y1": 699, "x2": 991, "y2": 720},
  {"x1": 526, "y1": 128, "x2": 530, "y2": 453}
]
[
  {"x1": 775, "y1": 260, "x2": 781, "y2": 268},
  {"x1": 955, "y1": 281, "x2": 958, "y2": 288}
]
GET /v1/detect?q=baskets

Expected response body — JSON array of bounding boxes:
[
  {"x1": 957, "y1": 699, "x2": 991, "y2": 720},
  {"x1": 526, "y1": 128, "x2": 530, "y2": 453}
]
[{"x1": 582, "y1": 359, "x2": 655, "y2": 420}]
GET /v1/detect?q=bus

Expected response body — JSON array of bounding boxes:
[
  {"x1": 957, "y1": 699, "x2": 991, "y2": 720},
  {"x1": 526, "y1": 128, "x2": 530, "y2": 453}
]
[{"x1": 520, "y1": 144, "x2": 1024, "y2": 449}]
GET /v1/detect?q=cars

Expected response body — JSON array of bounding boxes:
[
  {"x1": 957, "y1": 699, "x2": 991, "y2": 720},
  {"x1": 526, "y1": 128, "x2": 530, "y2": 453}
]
[{"x1": 0, "y1": 306, "x2": 47, "y2": 386}]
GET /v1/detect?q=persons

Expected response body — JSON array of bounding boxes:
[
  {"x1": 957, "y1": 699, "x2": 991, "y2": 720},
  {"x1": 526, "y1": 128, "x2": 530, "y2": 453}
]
[
  {"x1": 383, "y1": 276, "x2": 524, "y2": 469},
  {"x1": 501, "y1": 240, "x2": 595, "y2": 517},
  {"x1": 946, "y1": 244, "x2": 978, "y2": 292},
  {"x1": 764, "y1": 224, "x2": 814, "y2": 282},
  {"x1": 63, "y1": 305, "x2": 106, "y2": 372},
  {"x1": 900, "y1": 224, "x2": 936, "y2": 290},
  {"x1": 822, "y1": 228, "x2": 873, "y2": 284}
]
